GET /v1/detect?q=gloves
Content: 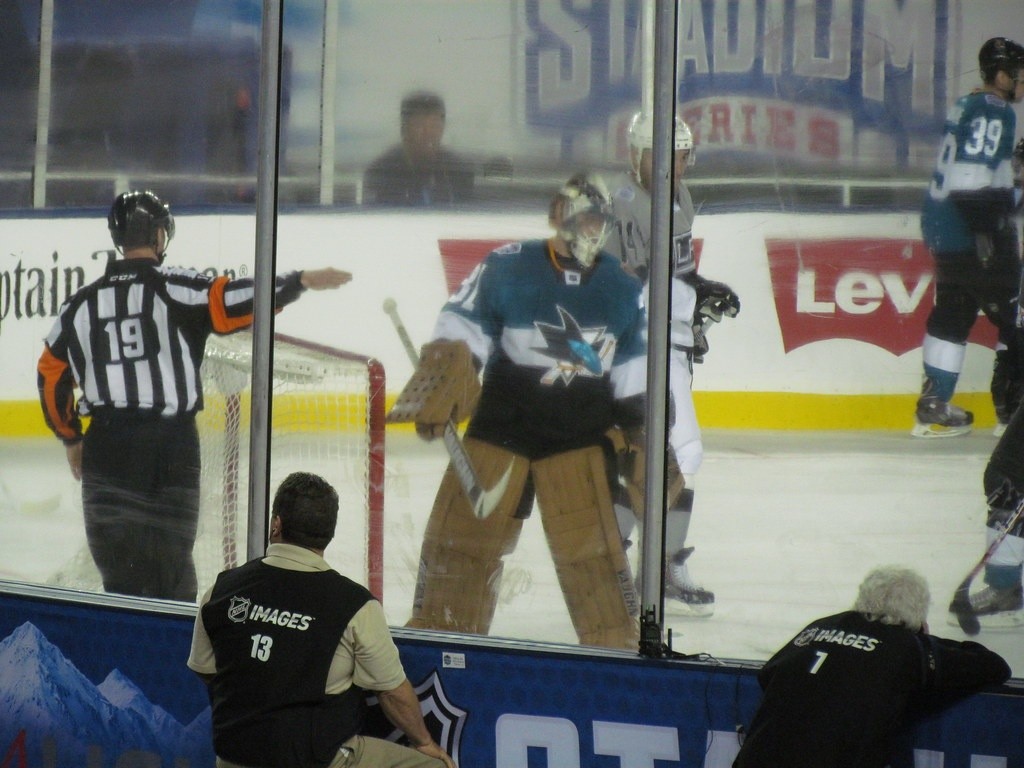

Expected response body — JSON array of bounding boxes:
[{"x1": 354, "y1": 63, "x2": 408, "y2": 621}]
[
  {"x1": 413, "y1": 408, "x2": 466, "y2": 440},
  {"x1": 694, "y1": 277, "x2": 740, "y2": 318}
]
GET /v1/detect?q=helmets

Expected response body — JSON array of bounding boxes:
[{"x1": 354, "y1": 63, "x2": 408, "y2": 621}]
[
  {"x1": 549, "y1": 170, "x2": 614, "y2": 270},
  {"x1": 627, "y1": 111, "x2": 696, "y2": 185},
  {"x1": 979, "y1": 37, "x2": 1024, "y2": 84},
  {"x1": 107, "y1": 190, "x2": 175, "y2": 245}
]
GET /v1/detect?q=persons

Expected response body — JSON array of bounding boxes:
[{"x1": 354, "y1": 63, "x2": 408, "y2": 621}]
[
  {"x1": 732, "y1": 567, "x2": 1013, "y2": 768},
  {"x1": 187, "y1": 470, "x2": 458, "y2": 767},
  {"x1": 911, "y1": 36, "x2": 1024, "y2": 439},
  {"x1": 591, "y1": 111, "x2": 742, "y2": 617},
  {"x1": 37, "y1": 190, "x2": 352, "y2": 604},
  {"x1": 947, "y1": 137, "x2": 1024, "y2": 615},
  {"x1": 362, "y1": 89, "x2": 474, "y2": 209},
  {"x1": 409, "y1": 173, "x2": 679, "y2": 652}
]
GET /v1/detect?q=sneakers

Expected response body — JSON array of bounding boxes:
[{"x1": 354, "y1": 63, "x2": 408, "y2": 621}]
[
  {"x1": 912, "y1": 373, "x2": 974, "y2": 437},
  {"x1": 947, "y1": 584, "x2": 1024, "y2": 629},
  {"x1": 633, "y1": 547, "x2": 715, "y2": 615},
  {"x1": 993, "y1": 403, "x2": 1013, "y2": 437}
]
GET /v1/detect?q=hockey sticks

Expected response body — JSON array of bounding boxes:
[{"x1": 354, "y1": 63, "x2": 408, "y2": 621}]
[
  {"x1": 691, "y1": 297, "x2": 732, "y2": 348},
  {"x1": 380, "y1": 295, "x2": 518, "y2": 521},
  {"x1": 953, "y1": 493, "x2": 1023, "y2": 638}
]
